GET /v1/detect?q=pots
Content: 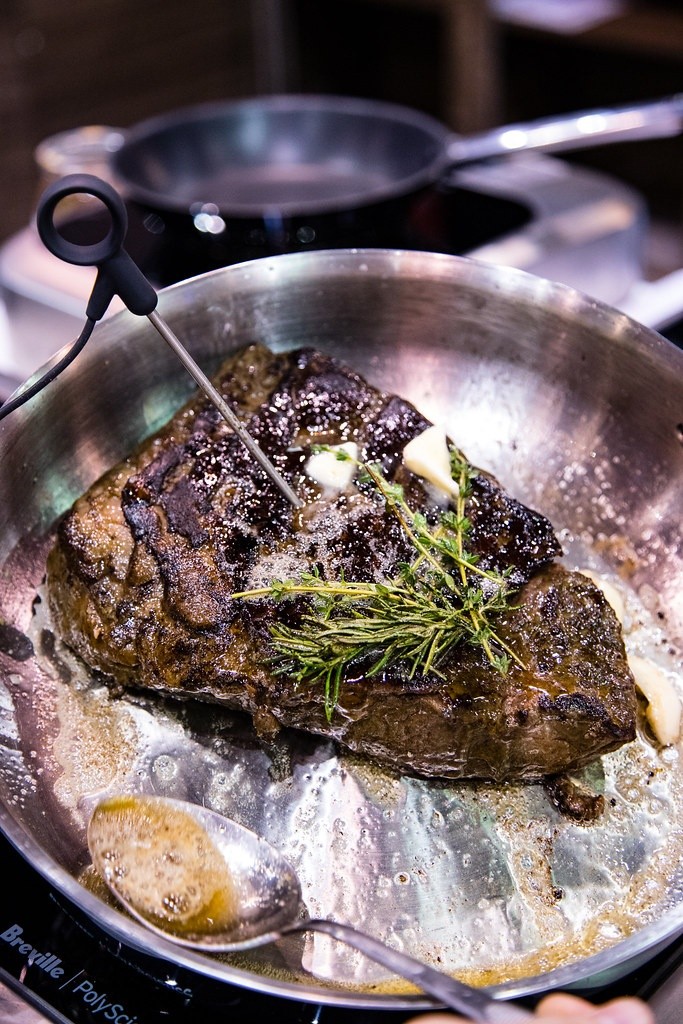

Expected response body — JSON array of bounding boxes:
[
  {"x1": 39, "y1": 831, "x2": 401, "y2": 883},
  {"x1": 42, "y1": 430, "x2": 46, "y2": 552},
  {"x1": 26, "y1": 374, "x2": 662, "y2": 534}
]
[{"x1": 109, "y1": 96, "x2": 683, "y2": 246}]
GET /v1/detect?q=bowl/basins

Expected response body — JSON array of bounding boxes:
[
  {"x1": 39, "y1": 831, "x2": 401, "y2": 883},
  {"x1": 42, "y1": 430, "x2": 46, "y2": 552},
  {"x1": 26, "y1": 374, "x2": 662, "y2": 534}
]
[{"x1": 0, "y1": 247, "x2": 683, "y2": 1000}]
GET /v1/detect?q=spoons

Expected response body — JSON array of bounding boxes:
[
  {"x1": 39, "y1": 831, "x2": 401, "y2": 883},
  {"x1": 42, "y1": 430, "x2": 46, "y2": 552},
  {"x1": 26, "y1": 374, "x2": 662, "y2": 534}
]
[{"x1": 91, "y1": 796, "x2": 539, "y2": 1024}]
